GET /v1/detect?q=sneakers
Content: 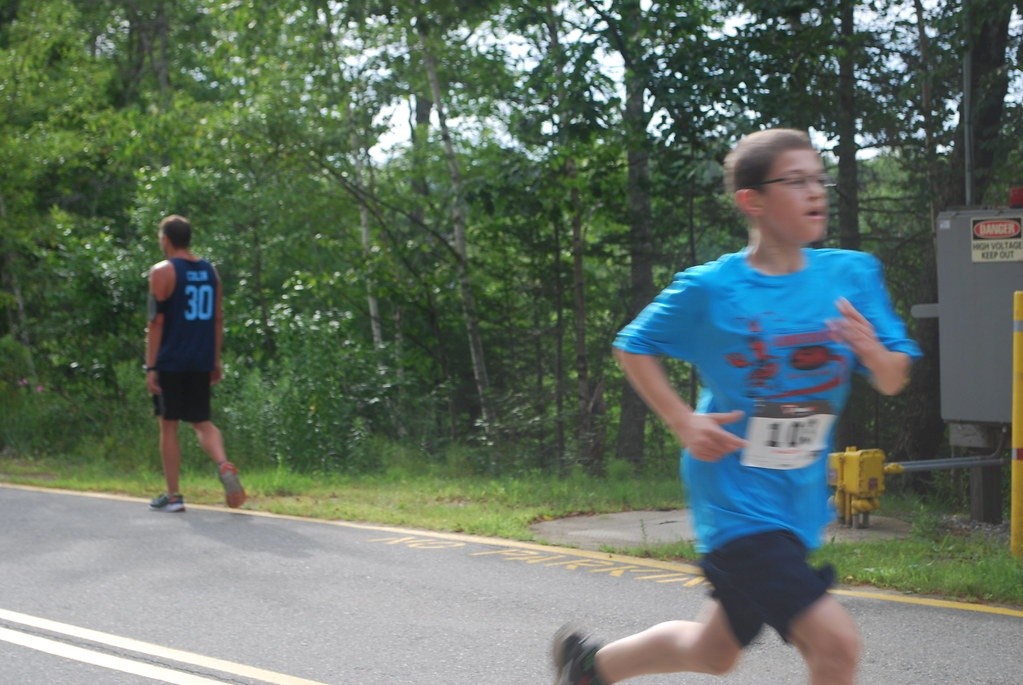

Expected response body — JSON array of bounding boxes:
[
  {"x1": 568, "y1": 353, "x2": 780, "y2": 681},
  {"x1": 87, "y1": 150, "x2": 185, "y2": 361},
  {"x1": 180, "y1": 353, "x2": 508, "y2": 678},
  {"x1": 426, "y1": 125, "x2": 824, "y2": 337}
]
[
  {"x1": 150, "y1": 494, "x2": 184, "y2": 512},
  {"x1": 219, "y1": 462, "x2": 245, "y2": 507}
]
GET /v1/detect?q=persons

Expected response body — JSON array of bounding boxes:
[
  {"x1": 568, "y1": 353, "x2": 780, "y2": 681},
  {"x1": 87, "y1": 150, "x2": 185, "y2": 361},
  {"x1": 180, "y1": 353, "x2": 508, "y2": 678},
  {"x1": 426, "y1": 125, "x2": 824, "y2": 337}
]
[
  {"x1": 552, "y1": 128, "x2": 925, "y2": 685},
  {"x1": 143, "y1": 215, "x2": 247, "y2": 513}
]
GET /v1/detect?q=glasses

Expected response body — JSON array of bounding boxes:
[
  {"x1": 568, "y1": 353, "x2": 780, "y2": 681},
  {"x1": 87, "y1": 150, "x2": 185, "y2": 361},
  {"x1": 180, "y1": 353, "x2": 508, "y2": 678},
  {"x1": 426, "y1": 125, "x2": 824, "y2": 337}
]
[{"x1": 747, "y1": 170, "x2": 836, "y2": 190}]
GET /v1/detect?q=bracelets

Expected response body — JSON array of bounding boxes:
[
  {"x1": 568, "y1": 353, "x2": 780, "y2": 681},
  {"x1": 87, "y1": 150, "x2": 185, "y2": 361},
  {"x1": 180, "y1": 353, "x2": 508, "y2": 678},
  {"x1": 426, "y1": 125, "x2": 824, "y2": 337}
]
[{"x1": 143, "y1": 365, "x2": 156, "y2": 371}]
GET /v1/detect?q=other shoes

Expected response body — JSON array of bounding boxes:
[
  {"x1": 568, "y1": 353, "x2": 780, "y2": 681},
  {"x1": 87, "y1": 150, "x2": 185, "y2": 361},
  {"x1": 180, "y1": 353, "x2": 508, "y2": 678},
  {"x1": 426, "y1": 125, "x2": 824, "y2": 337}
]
[{"x1": 555, "y1": 629, "x2": 597, "y2": 685}]
